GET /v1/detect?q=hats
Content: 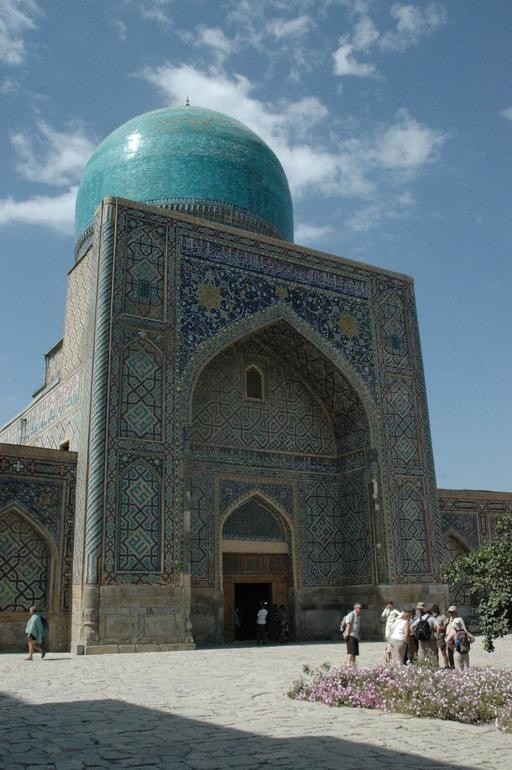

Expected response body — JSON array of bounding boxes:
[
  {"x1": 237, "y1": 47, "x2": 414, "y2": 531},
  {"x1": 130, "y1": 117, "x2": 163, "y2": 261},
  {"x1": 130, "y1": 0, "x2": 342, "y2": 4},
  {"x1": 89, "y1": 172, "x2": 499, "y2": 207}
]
[
  {"x1": 447, "y1": 606, "x2": 457, "y2": 612},
  {"x1": 415, "y1": 602, "x2": 441, "y2": 615}
]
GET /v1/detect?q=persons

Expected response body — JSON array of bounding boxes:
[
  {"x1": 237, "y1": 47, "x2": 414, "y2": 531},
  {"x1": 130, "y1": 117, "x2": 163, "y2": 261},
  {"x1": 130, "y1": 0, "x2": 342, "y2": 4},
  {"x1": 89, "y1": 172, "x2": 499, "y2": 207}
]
[
  {"x1": 381, "y1": 601, "x2": 475, "y2": 670},
  {"x1": 256, "y1": 601, "x2": 290, "y2": 644},
  {"x1": 339, "y1": 602, "x2": 362, "y2": 666},
  {"x1": 234, "y1": 607, "x2": 243, "y2": 642},
  {"x1": 24, "y1": 607, "x2": 48, "y2": 660}
]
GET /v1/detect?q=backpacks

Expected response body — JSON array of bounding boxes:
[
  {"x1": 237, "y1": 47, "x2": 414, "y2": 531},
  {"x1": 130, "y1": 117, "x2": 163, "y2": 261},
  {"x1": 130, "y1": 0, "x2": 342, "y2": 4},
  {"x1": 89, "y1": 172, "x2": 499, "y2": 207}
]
[
  {"x1": 455, "y1": 632, "x2": 470, "y2": 654},
  {"x1": 340, "y1": 616, "x2": 347, "y2": 632},
  {"x1": 416, "y1": 621, "x2": 431, "y2": 641},
  {"x1": 39, "y1": 614, "x2": 49, "y2": 630}
]
